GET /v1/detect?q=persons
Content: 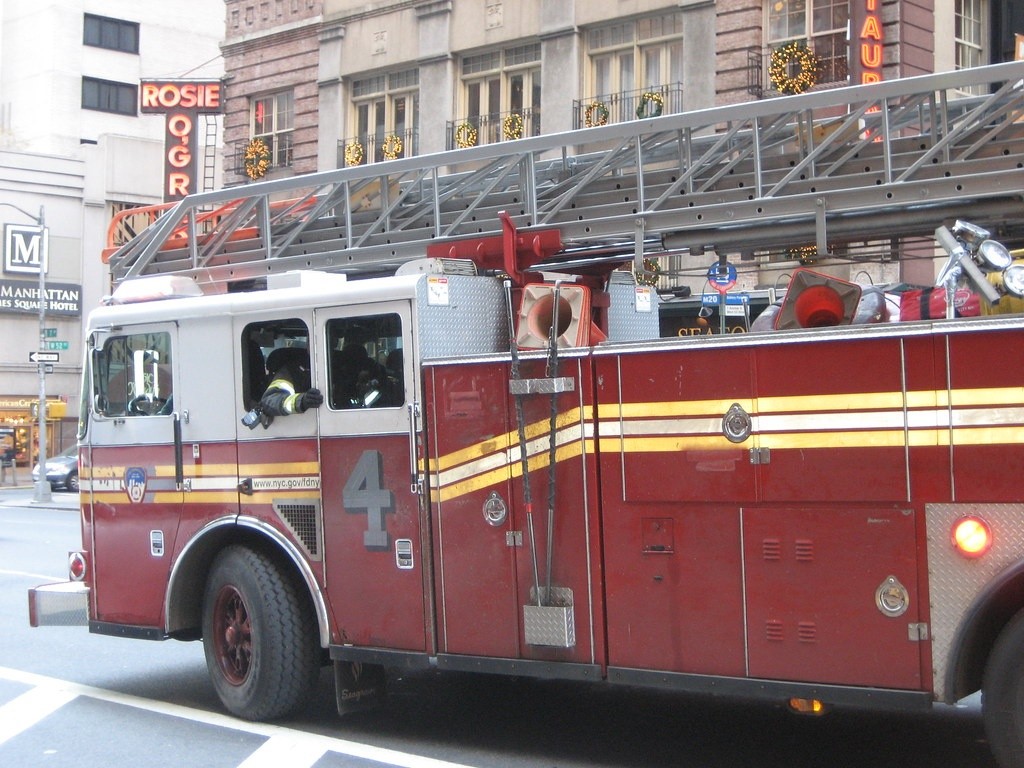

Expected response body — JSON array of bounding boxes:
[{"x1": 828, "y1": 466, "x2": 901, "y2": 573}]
[
  {"x1": 155, "y1": 392, "x2": 173, "y2": 415},
  {"x1": 261, "y1": 320, "x2": 396, "y2": 417},
  {"x1": 2, "y1": 444, "x2": 13, "y2": 460}
]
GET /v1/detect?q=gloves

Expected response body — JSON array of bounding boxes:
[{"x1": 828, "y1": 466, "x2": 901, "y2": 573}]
[{"x1": 300, "y1": 388, "x2": 323, "y2": 412}]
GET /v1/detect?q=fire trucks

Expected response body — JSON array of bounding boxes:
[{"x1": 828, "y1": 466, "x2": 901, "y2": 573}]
[{"x1": 27, "y1": 60, "x2": 1024, "y2": 768}]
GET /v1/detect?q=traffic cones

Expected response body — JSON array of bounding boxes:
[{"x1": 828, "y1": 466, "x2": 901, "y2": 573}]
[
  {"x1": 773, "y1": 265, "x2": 862, "y2": 334},
  {"x1": 514, "y1": 280, "x2": 608, "y2": 358}
]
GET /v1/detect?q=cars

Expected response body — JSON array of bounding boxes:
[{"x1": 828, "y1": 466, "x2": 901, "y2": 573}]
[{"x1": 31, "y1": 442, "x2": 80, "y2": 494}]
